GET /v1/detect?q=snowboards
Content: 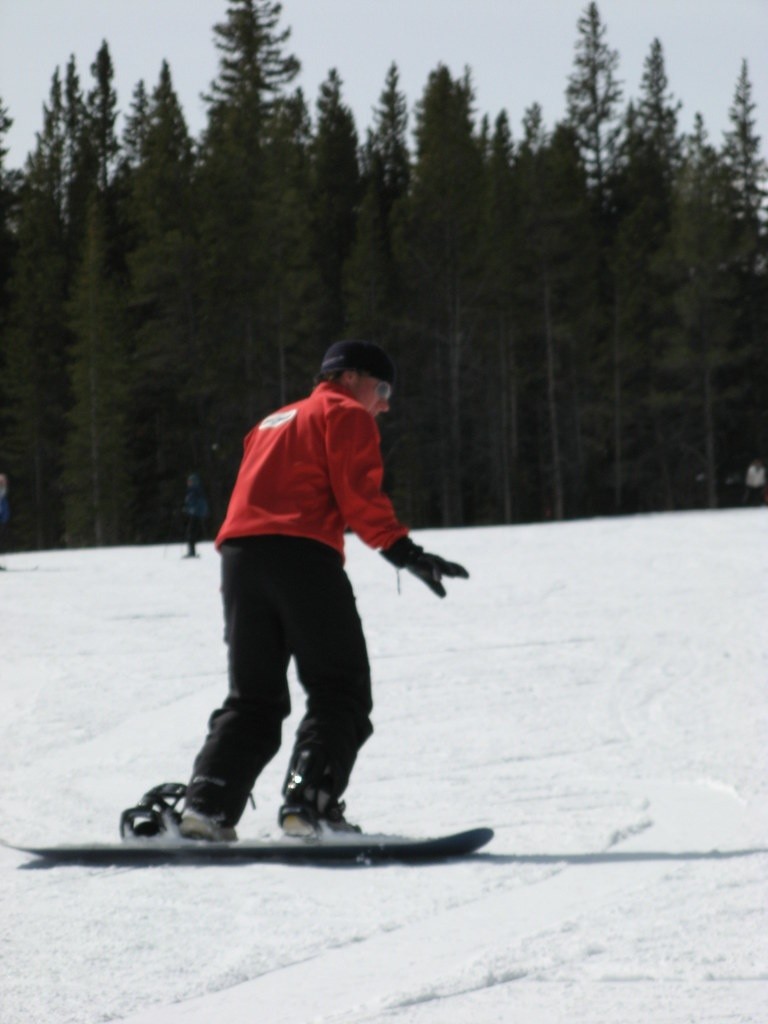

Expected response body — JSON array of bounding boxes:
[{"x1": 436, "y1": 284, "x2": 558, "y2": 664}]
[{"x1": 0, "y1": 821, "x2": 497, "y2": 870}]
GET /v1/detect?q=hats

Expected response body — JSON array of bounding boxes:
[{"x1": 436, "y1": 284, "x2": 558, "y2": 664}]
[{"x1": 314, "y1": 339, "x2": 395, "y2": 389}]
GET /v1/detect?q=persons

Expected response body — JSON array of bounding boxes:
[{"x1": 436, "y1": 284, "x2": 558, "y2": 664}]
[
  {"x1": 181, "y1": 343, "x2": 468, "y2": 841},
  {"x1": 746, "y1": 458, "x2": 766, "y2": 507}
]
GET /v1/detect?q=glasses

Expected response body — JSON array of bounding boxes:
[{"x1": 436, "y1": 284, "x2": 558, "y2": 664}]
[{"x1": 370, "y1": 378, "x2": 392, "y2": 402}]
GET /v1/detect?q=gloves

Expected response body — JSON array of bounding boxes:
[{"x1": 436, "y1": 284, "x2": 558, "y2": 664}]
[{"x1": 379, "y1": 537, "x2": 469, "y2": 598}]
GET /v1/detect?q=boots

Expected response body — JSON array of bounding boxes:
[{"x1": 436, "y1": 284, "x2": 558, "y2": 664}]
[
  {"x1": 179, "y1": 749, "x2": 262, "y2": 842},
  {"x1": 278, "y1": 742, "x2": 361, "y2": 842}
]
